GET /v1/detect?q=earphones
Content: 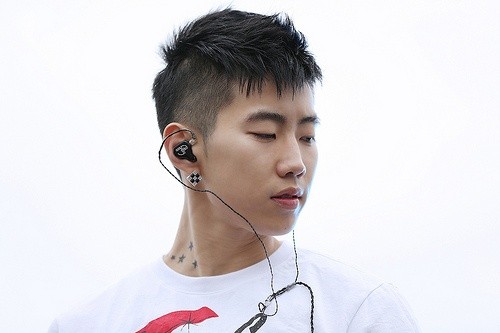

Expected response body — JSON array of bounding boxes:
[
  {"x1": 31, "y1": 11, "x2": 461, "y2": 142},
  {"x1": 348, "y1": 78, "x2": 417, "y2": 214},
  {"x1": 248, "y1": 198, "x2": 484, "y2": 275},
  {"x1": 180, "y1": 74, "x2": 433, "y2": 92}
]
[{"x1": 173, "y1": 133, "x2": 198, "y2": 162}]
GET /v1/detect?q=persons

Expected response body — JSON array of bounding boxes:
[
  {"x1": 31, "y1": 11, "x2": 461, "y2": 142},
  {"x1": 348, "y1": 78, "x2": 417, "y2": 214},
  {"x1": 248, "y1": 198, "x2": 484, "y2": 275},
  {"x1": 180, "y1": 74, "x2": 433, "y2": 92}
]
[{"x1": 49, "y1": 4, "x2": 428, "y2": 333}]
